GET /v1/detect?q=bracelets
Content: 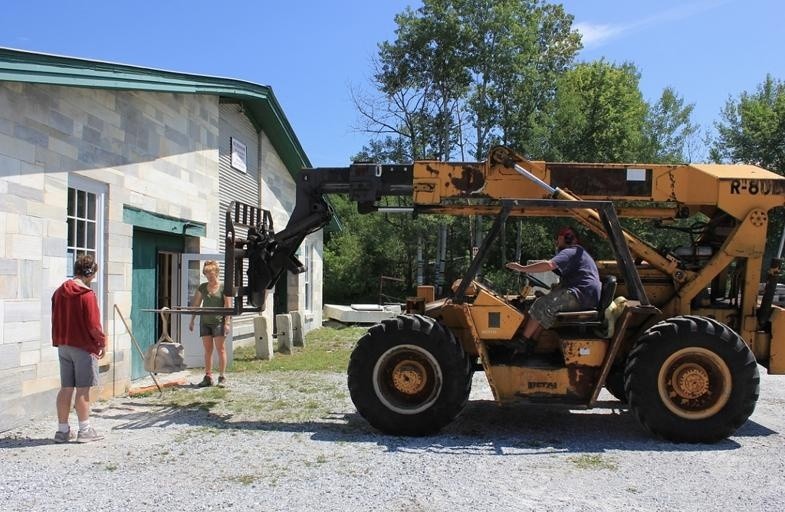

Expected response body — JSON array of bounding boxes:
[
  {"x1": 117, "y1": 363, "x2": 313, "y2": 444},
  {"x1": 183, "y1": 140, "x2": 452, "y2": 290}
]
[{"x1": 225, "y1": 321, "x2": 231, "y2": 325}]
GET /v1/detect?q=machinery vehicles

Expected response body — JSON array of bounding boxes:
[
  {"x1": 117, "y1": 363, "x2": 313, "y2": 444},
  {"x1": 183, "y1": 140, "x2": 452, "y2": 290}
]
[{"x1": 136, "y1": 137, "x2": 785, "y2": 446}]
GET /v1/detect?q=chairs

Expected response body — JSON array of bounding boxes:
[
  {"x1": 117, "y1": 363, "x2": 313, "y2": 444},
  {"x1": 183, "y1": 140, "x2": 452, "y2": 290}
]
[{"x1": 554, "y1": 275, "x2": 619, "y2": 328}]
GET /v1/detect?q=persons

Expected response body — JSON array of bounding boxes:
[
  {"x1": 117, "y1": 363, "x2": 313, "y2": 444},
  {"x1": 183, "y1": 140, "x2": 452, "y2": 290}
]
[
  {"x1": 504, "y1": 227, "x2": 603, "y2": 357},
  {"x1": 52, "y1": 253, "x2": 106, "y2": 443},
  {"x1": 188, "y1": 259, "x2": 233, "y2": 388}
]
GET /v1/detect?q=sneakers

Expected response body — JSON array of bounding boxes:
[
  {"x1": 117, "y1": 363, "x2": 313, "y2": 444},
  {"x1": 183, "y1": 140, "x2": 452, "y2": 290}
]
[
  {"x1": 216, "y1": 376, "x2": 226, "y2": 387},
  {"x1": 76, "y1": 428, "x2": 105, "y2": 443},
  {"x1": 500, "y1": 337, "x2": 539, "y2": 360},
  {"x1": 198, "y1": 378, "x2": 213, "y2": 388},
  {"x1": 55, "y1": 428, "x2": 77, "y2": 443}
]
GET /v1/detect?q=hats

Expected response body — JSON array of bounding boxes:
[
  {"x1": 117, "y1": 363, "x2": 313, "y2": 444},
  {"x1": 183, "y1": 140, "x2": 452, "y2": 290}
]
[{"x1": 557, "y1": 227, "x2": 583, "y2": 244}]
